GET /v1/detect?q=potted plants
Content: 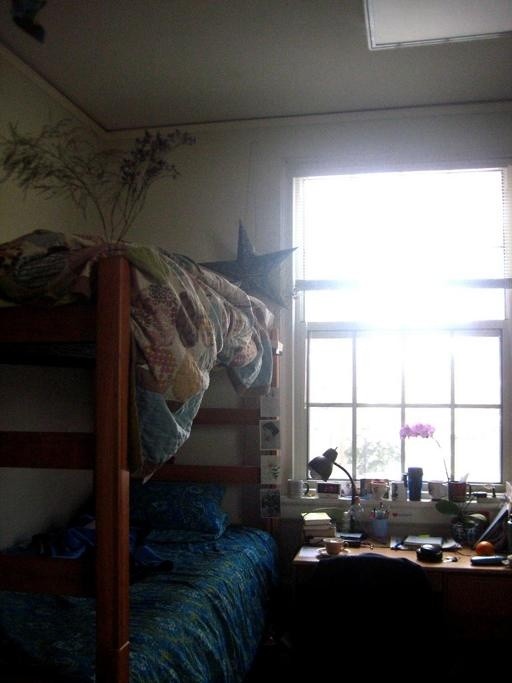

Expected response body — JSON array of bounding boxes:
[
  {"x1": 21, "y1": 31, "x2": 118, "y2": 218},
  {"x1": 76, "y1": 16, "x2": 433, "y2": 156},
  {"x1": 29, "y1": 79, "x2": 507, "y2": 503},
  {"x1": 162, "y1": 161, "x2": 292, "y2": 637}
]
[{"x1": 435, "y1": 496, "x2": 489, "y2": 547}]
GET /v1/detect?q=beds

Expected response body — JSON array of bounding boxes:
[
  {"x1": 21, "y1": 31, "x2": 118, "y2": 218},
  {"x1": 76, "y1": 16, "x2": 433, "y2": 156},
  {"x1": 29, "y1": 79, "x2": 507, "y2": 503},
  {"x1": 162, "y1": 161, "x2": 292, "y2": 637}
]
[{"x1": 1, "y1": 255, "x2": 283, "y2": 683}]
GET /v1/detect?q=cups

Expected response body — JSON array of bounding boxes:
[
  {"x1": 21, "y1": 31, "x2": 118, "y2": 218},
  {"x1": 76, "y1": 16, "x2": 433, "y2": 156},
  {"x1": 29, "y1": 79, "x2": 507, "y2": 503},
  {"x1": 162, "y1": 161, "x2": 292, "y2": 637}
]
[
  {"x1": 323, "y1": 537, "x2": 349, "y2": 555},
  {"x1": 448, "y1": 480, "x2": 472, "y2": 503},
  {"x1": 371, "y1": 481, "x2": 389, "y2": 500},
  {"x1": 287, "y1": 478, "x2": 309, "y2": 499},
  {"x1": 402, "y1": 466, "x2": 423, "y2": 501},
  {"x1": 428, "y1": 479, "x2": 448, "y2": 501}
]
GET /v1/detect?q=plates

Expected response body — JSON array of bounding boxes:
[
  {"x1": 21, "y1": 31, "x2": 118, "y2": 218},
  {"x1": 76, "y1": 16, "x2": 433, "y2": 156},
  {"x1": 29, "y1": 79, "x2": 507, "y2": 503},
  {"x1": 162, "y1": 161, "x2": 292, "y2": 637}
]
[{"x1": 316, "y1": 547, "x2": 351, "y2": 557}]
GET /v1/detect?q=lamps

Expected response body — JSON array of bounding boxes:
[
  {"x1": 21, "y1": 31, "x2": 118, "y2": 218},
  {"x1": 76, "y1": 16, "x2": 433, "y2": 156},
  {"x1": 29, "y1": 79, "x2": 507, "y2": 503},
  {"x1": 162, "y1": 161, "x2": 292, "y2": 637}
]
[{"x1": 308, "y1": 449, "x2": 361, "y2": 534}]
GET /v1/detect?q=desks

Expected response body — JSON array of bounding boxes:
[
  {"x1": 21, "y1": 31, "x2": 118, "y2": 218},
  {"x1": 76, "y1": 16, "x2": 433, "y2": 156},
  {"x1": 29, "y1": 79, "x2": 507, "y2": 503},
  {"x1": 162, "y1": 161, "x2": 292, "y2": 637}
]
[{"x1": 292, "y1": 535, "x2": 512, "y2": 683}]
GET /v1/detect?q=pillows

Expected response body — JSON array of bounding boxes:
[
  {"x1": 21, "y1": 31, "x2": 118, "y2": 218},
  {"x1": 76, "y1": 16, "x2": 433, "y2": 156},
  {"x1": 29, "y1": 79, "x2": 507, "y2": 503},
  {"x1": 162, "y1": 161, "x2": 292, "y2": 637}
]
[{"x1": 128, "y1": 477, "x2": 228, "y2": 542}]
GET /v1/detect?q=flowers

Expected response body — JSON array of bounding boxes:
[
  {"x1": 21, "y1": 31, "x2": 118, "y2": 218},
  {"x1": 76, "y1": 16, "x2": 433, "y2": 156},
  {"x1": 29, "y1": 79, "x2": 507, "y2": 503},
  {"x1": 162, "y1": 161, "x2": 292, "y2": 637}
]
[{"x1": 400, "y1": 424, "x2": 450, "y2": 483}]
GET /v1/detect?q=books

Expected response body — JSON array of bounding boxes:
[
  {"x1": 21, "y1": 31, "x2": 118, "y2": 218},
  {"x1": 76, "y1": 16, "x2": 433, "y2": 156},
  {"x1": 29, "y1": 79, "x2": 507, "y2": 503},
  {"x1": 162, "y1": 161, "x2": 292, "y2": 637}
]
[
  {"x1": 301, "y1": 512, "x2": 333, "y2": 524},
  {"x1": 403, "y1": 534, "x2": 443, "y2": 547},
  {"x1": 302, "y1": 522, "x2": 333, "y2": 529}
]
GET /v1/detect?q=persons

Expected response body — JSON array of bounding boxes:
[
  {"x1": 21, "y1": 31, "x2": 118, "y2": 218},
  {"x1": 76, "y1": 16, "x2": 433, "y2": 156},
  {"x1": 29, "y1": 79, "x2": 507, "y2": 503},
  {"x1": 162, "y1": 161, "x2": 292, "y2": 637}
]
[{"x1": 262, "y1": 421, "x2": 280, "y2": 448}]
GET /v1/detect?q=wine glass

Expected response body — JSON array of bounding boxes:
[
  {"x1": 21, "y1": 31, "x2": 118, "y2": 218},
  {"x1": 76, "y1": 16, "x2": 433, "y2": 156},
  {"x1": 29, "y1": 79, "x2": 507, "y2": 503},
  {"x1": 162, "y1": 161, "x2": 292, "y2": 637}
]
[{"x1": 389, "y1": 481, "x2": 407, "y2": 502}]
